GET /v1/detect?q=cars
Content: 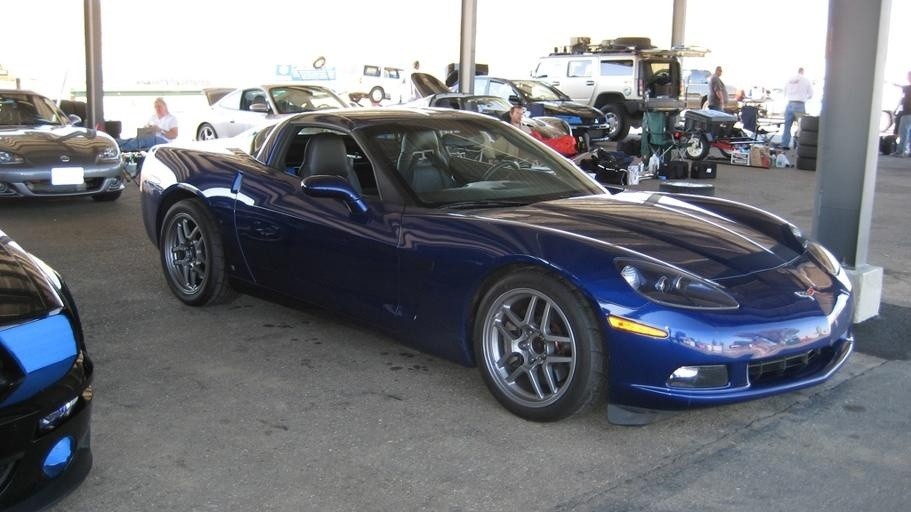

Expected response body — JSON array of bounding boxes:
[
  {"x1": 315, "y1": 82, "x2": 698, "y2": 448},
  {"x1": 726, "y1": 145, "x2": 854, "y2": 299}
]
[
  {"x1": 404, "y1": 72, "x2": 577, "y2": 158},
  {"x1": 139, "y1": 105, "x2": 857, "y2": 425},
  {"x1": 0, "y1": 230, "x2": 94, "y2": 512},
  {"x1": 447, "y1": 76, "x2": 611, "y2": 144},
  {"x1": 193, "y1": 82, "x2": 347, "y2": 143},
  {"x1": 0, "y1": 89, "x2": 126, "y2": 202},
  {"x1": 655, "y1": 68, "x2": 738, "y2": 103}
]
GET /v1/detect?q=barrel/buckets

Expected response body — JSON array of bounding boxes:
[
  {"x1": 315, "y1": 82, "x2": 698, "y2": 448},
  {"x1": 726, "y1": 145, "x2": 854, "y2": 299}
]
[
  {"x1": 649, "y1": 153, "x2": 660, "y2": 175},
  {"x1": 775, "y1": 150, "x2": 785, "y2": 168}
]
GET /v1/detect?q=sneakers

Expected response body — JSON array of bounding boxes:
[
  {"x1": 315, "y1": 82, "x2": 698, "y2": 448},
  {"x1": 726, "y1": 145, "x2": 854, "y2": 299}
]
[{"x1": 890, "y1": 152, "x2": 911, "y2": 158}]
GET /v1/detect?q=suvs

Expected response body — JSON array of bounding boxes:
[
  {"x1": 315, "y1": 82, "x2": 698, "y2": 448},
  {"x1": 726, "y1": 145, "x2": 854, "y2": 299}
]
[
  {"x1": 348, "y1": 57, "x2": 410, "y2": 106},
  {"x1": 530, "y1": 35, "x2": 711, "y2": 142}
]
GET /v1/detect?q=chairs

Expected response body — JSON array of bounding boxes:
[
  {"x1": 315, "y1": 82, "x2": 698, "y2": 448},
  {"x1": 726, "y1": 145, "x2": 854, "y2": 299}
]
[
  {"x1": 395, "y1": 126, "x2": 454, "y2": 188},
  {"x1": 298, "y1": 130, "x2": 363, "y2": 196}
]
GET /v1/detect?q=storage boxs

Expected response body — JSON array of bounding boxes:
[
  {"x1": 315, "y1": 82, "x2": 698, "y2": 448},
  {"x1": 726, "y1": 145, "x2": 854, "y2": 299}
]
[{"x1": 684, "y1": 109, "x2": 737, "y2": 139}]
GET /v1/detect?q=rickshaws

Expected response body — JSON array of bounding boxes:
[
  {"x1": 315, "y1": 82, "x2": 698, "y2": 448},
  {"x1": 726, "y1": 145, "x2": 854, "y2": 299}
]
[{"x1": 671, "y1": 118, "x2": 766, "y2": 160}]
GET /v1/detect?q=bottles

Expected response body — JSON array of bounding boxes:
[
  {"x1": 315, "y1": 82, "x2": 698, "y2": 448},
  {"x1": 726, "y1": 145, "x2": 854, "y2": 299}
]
[{"x1": 647, "y1": 154, "x2": 659, "y2": 180}]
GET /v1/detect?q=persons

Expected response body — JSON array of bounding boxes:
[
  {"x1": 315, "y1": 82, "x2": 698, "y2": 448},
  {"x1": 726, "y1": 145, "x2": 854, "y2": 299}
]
[
  {"x1": 145, "y1": 97, "x2": 179, "y2": 144},
  {"x1": 891, "y1": 71, "x2": 910, "y2": 158},
  {"x1": 708, "y1": 66, "x2": 729, "y2": 114},
  {"x1": 781, "y1": 67, "x2": 813, "y2": 151},
  {"x1": 509, "y1": 106, "x2": 533, "y2": 136}
]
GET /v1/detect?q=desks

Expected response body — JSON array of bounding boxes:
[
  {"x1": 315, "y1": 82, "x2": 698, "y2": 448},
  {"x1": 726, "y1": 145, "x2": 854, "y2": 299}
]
[{"x1": 121, "y1": 149, "x2": 141, "y2": 188}]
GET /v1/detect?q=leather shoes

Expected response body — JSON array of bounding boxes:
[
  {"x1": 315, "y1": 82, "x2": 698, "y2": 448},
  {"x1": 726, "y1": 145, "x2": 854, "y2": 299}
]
[{"x1": 782, "y1": 147, "x2": 791, "y2": 150}]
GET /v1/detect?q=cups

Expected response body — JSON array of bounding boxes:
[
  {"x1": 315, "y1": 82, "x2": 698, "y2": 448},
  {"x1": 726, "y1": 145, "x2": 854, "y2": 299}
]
[{"x1": 627, "y1": 165, "x2": 639, "y2": 185}]
